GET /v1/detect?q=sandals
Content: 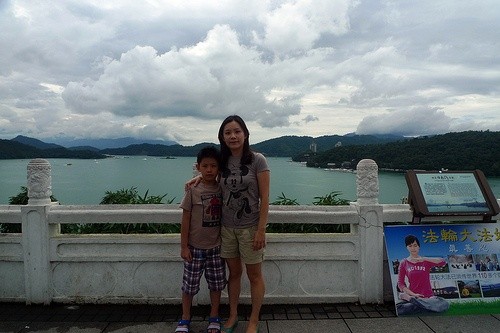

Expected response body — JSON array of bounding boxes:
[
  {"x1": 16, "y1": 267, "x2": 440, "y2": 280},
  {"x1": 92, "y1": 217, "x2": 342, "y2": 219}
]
[
  {"x1": 221, "y1": 320, "x2": 238, "y2": 333},
  {"x1": 174, "y1": 320, "x2": 190, "y2": 333},
  {"x1": 207, "y1": 318, "x2": 221, "y2": 333}
]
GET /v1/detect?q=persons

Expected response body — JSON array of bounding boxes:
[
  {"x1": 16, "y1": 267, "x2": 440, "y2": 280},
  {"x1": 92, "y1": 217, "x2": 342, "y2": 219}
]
[
  {"x1": 174, "y1": 144, "x2": 227, "y2": 333},
  {"x1": 475, "y1": 255, "x2": 500, "y2": 271},
  {"x1": 394, "y1": 235, "x2": 449, "y2": 315},
  {"x1": 184, "y1": 114, "x2": 270, "y2": 333}
]
